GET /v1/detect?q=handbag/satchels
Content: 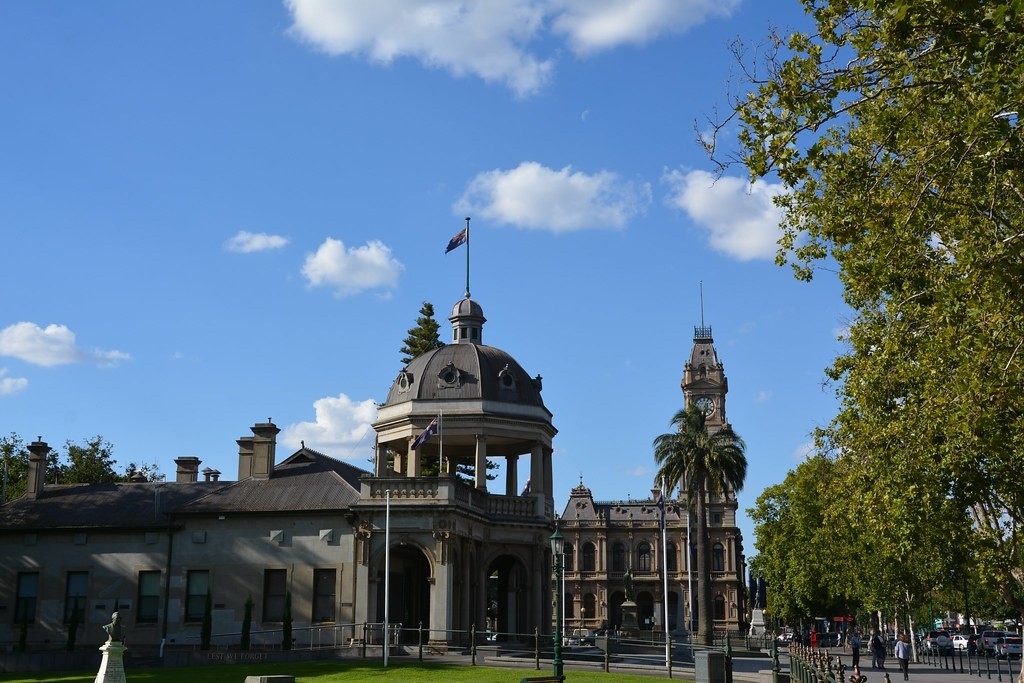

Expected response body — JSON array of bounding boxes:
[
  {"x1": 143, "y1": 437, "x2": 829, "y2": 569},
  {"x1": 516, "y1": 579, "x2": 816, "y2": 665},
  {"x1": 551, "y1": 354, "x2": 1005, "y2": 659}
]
[{"x1": 867, "y1": 640, "x2": 872, "y2": 650}]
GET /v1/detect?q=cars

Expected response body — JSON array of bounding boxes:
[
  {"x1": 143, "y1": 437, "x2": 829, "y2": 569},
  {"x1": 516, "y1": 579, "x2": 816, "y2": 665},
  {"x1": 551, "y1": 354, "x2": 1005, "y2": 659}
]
[
  {"x1": 967, "y1": 635, "x2": 981, "y2": 653},
  {"x1": 889, "y1": 634, "x2": 895, "y2": 640},
  {"x1": 993, "y1": 637, "x2": 1023, "y2": 659},
  {"x1": 777, "y1": 633, "x2": 793, "y2": 641},
  {"x1": 951, "y1": 635, "x2": 970, "y2": 650}
]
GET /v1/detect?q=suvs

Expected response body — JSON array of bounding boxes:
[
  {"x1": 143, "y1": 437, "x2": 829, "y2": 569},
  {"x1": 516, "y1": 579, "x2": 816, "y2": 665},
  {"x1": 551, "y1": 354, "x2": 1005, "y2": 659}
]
[
  {"x1": 921, "y1": 630, "x2": 953, "y2": 655},
  {"x1": 975, "y1": 631, "x2": 1007, "y2": 655}
]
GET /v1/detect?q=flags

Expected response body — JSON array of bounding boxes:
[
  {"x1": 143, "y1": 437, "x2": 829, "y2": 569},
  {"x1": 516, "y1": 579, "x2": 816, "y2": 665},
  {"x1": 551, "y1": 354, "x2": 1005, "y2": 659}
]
[
  {"x1": 444, "y1": 227, "x2": 466, "y2": 255},
  {"x1": 655, "y1": 483, "x2": 665, "y2": 532},
  {"x1": 410, "y1": 418, "x2": 437, "y2": 450}
]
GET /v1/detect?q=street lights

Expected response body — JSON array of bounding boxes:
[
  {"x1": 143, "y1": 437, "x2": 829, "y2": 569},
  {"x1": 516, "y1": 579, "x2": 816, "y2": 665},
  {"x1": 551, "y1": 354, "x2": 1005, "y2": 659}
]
[{"x1": 548, "y1": 523, "x2": 566, "y2": 676}]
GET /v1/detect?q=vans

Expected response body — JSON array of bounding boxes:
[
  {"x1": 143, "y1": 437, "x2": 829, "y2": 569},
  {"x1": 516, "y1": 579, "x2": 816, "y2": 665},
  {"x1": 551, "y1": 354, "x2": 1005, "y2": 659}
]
[{"x1": 816, "y1": 633, "x2": 843, "y2": 647}]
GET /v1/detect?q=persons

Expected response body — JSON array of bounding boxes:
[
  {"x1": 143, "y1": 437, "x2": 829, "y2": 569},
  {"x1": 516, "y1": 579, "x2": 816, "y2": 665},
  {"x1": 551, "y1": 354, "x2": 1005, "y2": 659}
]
[
  {"x1": 771, "y1": 630, "x2": 775, "y2": 639},
  {"x1": 122, "y1": 636, "x2": 128, "y2": 666},
  {"x1": 925, "y1": 627, "x2": 931, "y2": 638},
  {"x1": 851, "y1": 633, "x2": 861, "y2": 668},
  {"x1": 836, "y1": 629, "x2": 843, "y2": 647},
  {"x1": 809, "y1": 623, "x2": 817, "y2": 650},
  {"x1": 101, "y1": 612, "x2": 126, "y2": 642},
  {"x1": 872, "y1": 629, "x2": 888, "y2": 670},
  {"x1": 894, "y1": 632, "x2": 912, "y2": 681},
  {"x1": 752, "y1": 626, "x2": 757, "y2": 635},
  {"x1": 623, "y1": 569, "x2": 635, "y2": 602}
]
